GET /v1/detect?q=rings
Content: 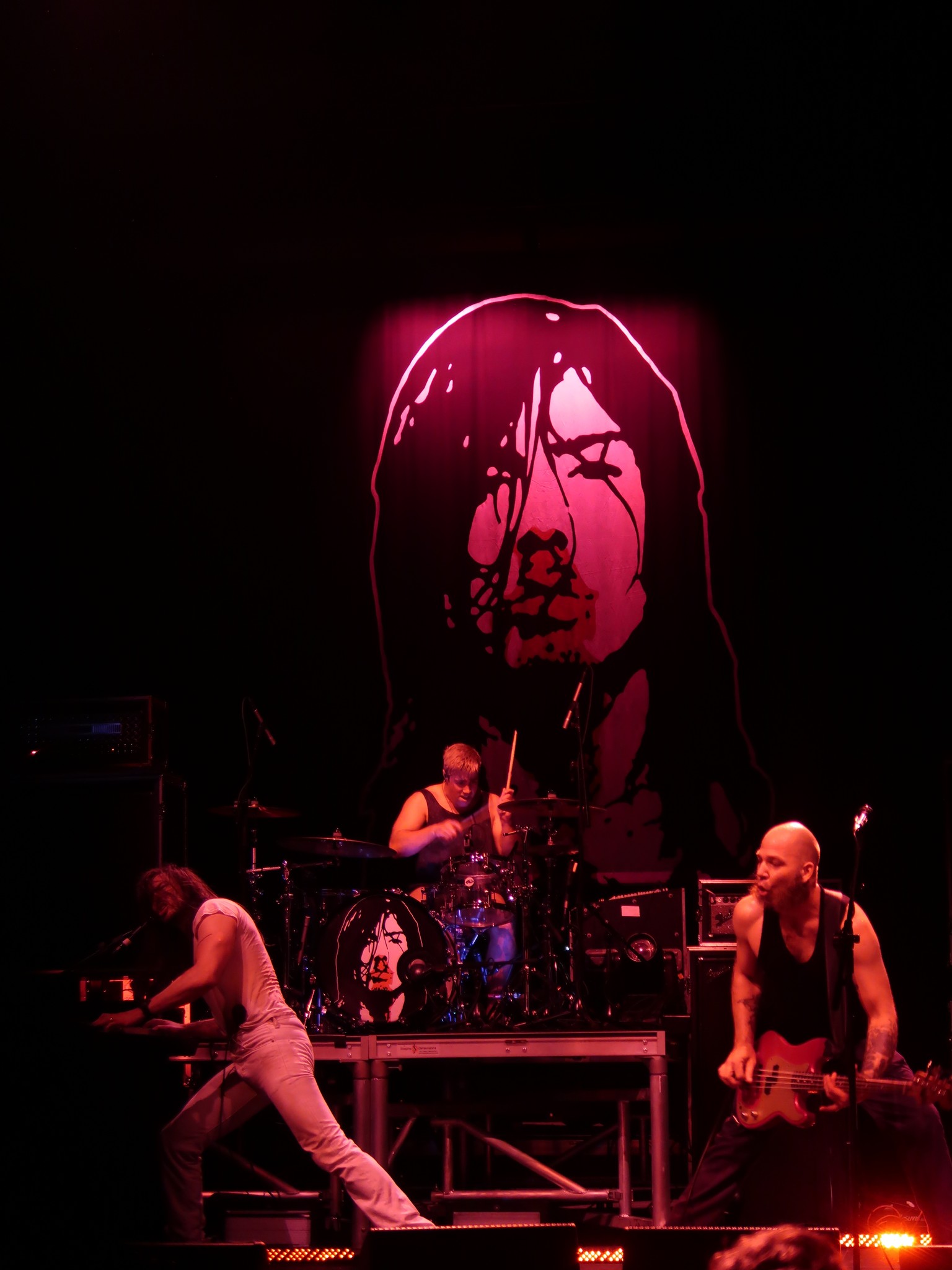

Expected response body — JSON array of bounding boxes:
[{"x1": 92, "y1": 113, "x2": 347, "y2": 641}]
[{"x1": 110, "y1": 1018, "x2": 113, "y2": 1020}]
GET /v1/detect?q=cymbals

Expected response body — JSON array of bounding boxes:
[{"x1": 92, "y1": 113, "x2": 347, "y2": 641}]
[
  {"x1": 527, "y1": 845, "x2": 579, "y2": 857},
  {"x1": 281, "y1": 835, "x2": 398, "y2": 860},
  {"x1": 496, "y1": 797, "x2": 591, "y2": 817},
  {"x1": 204, "y1": 803, "x2": 300, "y2": 820}
]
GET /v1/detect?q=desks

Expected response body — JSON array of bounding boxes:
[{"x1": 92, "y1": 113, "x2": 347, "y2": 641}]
[{"x1": 168, "y1": 1029, "x2": 670, "y2": 1259}]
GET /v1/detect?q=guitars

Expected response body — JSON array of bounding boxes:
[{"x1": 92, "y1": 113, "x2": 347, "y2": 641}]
[{"x1": 735, "y1": 1028, "x2": 952, "y2": 1131}]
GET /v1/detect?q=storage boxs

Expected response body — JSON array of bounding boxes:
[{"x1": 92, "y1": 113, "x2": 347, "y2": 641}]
[{"x1": 568, "y1": 884, "x2": 692, "y2": 1025}]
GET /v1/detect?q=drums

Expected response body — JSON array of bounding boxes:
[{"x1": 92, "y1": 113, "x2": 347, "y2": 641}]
[
  {"x1": 434, "y1": 850, "x2": 518, "y2": 929},
  {"x1": 317, "y1": 887, "x2": 369, "y2": 926},
  {"x1": 309, "y1": 890, "x2": 462, "y2": 1035}
]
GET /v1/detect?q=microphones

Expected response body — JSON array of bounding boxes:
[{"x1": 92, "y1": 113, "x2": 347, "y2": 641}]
[
  {"x1": 562, "y1": 669, "x2": 587, "y2": 729},
  {"x1": 116, "y1": 915, "x2": 155, "y2": 952},
  {"x1": 253, "y1": 708, "x2": 276, "y2": 745}
]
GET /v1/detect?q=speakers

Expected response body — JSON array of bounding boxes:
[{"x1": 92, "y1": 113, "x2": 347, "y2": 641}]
[
  {"x1": 141, "y1": 1241, "x2": 270, "y2": 1270},
  {"x1": 623, "y1": 1227, "x2": 841, "y2": 1270},
  {"x1": 350, "y1": 1223, "x2": 579, "y2": 1270}
]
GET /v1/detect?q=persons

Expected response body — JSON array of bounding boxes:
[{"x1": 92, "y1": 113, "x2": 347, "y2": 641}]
[
  {"x1": 137, "y1": 1002, "x2": 153, "y2": 1020},
  {"x1": 94, "y1": 864, "x2": 437, "y2": 1242},
  {"x1": 669, "y1": 820, "x2": 952, "y2": 1231},
  {"x1": 708, "y1": 1222, "x2": 846, "y2": 1270},
  {"x1": 387, "y1": 742, "x2": 516, "y2": 1025}
]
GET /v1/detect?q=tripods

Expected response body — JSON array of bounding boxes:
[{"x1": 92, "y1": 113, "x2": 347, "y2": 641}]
[{"x1": 233, "y1": 701, "x2": 655, "y2": 1031}]
